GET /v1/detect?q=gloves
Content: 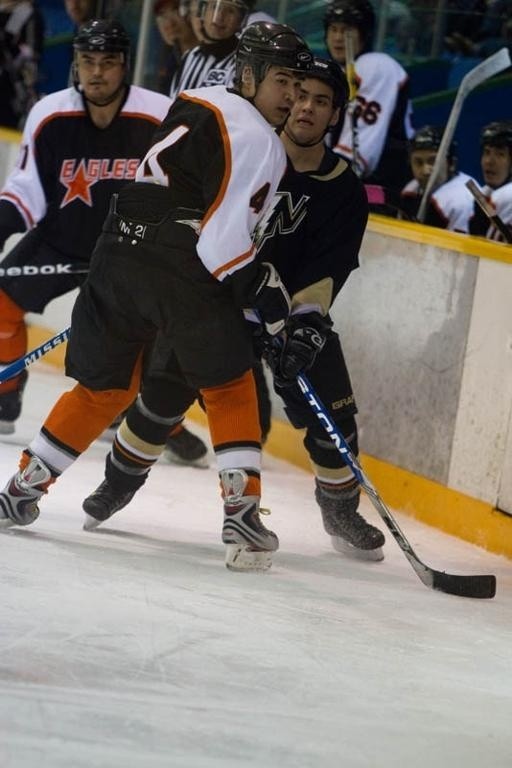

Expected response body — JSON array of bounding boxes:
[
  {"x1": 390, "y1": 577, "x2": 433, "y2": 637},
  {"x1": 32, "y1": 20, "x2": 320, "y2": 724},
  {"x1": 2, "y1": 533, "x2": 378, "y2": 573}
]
[
  {"x1": 275, "y1": 306, "x2": 333, "y2": 392},
  {"x1": 248, "y1": 258, "x2": 295, "y2": 350}
]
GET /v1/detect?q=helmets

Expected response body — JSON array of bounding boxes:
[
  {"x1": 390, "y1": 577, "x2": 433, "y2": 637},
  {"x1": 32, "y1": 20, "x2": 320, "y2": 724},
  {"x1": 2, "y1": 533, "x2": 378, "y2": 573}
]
[
  {"x1": 235, "y1": 20, "x2": 315, "y2": 82},
  {"x1": 73, "y1": 16, "x2": 134, "y2": 86},
  {"x1": 407, "y1": 125, "x2": 459, "y2": 172},
  {"x1": 479, "y1": 119, "x2": 512, "y2": 148},
  {"x1": 196, "y1": 1, "x2": 259, "y2": 29},
  {"x1": 324, "y1": 1, "x2": 377, "y2": 41},
  {"x1": 308, "y1": 54, "x2": 351, "y2": 110}
]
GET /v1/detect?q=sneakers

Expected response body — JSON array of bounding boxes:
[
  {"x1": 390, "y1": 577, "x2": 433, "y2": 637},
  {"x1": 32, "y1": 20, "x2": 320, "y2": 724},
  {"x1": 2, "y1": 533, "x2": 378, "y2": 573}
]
[
  {"x1": 223, "y1": 500, "x2": 280, "y2": 553},
  {"x1": 167, "y1": 416, "x2": 209, "y2": 462},
  {"x1": 321, "y1": 505, "x2": 386, "y2": 551},
  {"x1": 83, "y1": 477, "x2": 138, "y2": 522},
  {"x1": 1, "y1": 471, "x2": 47, "y2": 530},
  {"x1": 2, "y1": 370, "x2": 28, "y2": 422}
]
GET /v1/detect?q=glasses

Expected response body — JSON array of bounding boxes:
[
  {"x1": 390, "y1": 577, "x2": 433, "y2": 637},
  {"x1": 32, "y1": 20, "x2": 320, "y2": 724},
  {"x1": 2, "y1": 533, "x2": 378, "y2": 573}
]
[{"x1": 156, "y1": 10, "x2": 179, "y2": 25}]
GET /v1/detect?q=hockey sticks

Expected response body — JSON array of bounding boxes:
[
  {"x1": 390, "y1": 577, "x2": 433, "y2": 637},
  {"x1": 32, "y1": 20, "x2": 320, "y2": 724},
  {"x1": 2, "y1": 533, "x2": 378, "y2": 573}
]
[
  {"x1": 250, "y1": 310, "x2": 496, "y2": 598},
  {"x1": 417, "y1": 47, "x2": 512, "y2": 227}
]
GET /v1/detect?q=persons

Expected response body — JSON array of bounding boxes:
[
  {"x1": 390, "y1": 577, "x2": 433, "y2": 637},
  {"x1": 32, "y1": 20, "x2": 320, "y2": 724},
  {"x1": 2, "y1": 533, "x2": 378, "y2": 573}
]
[
  {"x1": 0, "y1": 21, "x2": 207, "y2": 463},
  {"x1": 2, "y1": 2, "x2": 512, "y2": 247},
  {"x1": 82, "y1": 56, "x2": 389, "y2": 562},
  {"x1": 0, "y1": 22, "x2": 312, "y2": 571}
]
